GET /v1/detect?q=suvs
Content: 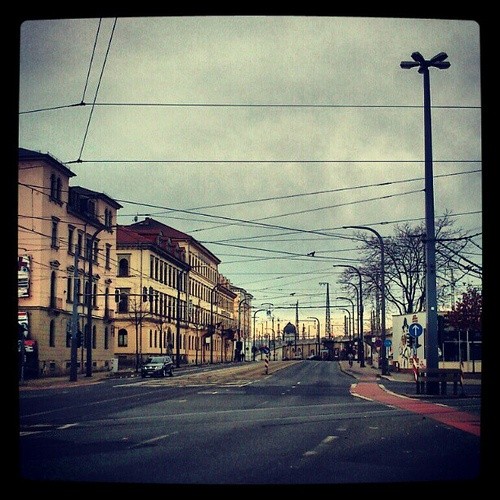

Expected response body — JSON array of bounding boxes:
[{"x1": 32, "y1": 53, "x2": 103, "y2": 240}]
[{"x1": 141, "y1": 356, "x2": 175, "y2": 377}]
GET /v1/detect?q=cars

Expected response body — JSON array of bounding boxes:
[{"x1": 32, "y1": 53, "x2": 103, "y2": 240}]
[{"x1": 307, "y1": 354, "x2": 320, "y2": 360}]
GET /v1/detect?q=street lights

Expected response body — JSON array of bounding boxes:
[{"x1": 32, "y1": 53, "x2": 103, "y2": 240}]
[
  {"x1": 253, "y1": 308, "x2": 266, "y2": 361},
  {"x1": 341, "y1": 225, "x2": 386, "y2": 375},
  {"x1": 210, "y1": 282, "x2": 228, "y2": 363},
  {"x1": 86, "y1": 224, "x2": 108, "y2": 377},
  {"x1": 332, "y1": 264, "x2": 365, "y2": 368},
  {"x1": 400, "y1": 50, "x2": 454, "y2": 396},
  {"x1": 335, "y1": 296, "x2": 354, "y2": 350},
  {"x1": 238, "y1": 298, "x2": 250, "y2": 341},
  {"x1": 175, "y1": 266, "x2": 197, "y2": 368}
]
